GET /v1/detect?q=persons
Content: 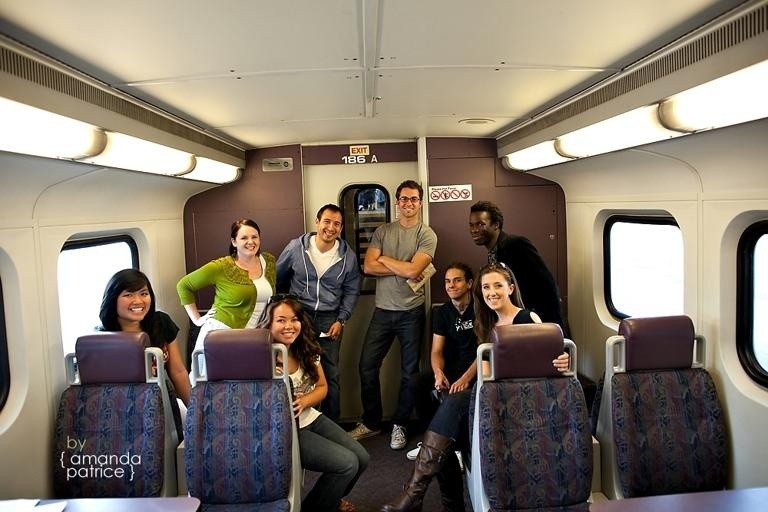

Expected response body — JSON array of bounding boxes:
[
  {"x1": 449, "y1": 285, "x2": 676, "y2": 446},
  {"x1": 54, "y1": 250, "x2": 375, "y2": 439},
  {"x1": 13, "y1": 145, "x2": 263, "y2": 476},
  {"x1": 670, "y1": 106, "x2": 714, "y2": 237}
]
[
  {"x1": 467, "y1": 199, "x2": 569, "y2": 338},
  {"x1": 346, "y1": 179, "x2": 437, "y2": 450},
  {"x1": 175, "y1": 218, "x2": 278, "y2": 387},
  {"x1": 276, "y1": 203, "x2": 363, "y2": 424},
  {"x1": 380, "y1": 261, "x2": 570, "y2": 512},
  {"x1": 255, "y1": 293, "x2": 370, "y2": 512},
  {"x1": 406, "y1": 262, "x2": 479, "y2": 472},
  {"x1": 94, "y1": 268, "x2": 193, "y2": 409}
]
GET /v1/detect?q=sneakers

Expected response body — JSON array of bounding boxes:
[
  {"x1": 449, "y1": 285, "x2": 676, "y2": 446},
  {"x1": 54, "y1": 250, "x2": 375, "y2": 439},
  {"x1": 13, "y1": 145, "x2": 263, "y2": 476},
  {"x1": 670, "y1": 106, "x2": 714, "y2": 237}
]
[
  {"x1": 347, "y1": 422, "x2": 382, "y2": 440},
  {"x1": 406, "y1": 441, "x2": 423, "y2": 461},
  {"x1": 390, "y1": 424, "x2": 407, "y2": 449}
]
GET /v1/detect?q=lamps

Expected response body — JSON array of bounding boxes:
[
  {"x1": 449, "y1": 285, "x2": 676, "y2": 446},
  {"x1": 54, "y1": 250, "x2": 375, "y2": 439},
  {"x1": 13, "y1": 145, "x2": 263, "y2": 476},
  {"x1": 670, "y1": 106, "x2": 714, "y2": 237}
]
[
  {"x1": 74, "y1": 129, "x2": 196, "y2": 177},
  {"x1": 656, "y1": 58, "x2": 768, "y2": 135},
  {"x1": 0, "y1": 96, "x2": 106, "y2": 161},
  {"x1": 554, "y1": 101, "x2": 662, "y2": 160},
  {"x1": 501, "y1": 140, "x2": 578, "y2": 173},
  {"x1": 173, "y1": 155, "x2": 242, "y2": 185}
]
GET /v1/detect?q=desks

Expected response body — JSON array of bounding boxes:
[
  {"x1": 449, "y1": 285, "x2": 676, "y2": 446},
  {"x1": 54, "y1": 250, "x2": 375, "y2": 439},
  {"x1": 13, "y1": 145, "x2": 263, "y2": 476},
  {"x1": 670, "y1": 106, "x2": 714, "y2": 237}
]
[
  {"x1": 2, "y1": 498, "x2": 202, "y2": 512},
  {"x1": 589, "y1": 487, "x2": 768, "y2": 512}
]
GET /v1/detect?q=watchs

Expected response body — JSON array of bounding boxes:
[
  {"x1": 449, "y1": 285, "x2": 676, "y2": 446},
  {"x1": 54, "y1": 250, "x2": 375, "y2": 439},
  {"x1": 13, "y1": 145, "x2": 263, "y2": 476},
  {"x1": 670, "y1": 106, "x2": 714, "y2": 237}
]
[{"x1": 335, "y1": 317, "x2": 346, "y2": 326}]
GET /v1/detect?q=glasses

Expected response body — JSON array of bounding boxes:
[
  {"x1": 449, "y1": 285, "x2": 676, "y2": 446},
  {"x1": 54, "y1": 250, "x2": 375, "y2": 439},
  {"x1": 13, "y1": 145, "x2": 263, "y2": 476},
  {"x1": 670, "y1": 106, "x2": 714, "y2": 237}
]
[
  {"x1": 399, "y1": 196, "x2": 420, "y2": 203},
  {"x1": 267, "y1": 293, "x2": 298, "y2": 305},
  {"x1": 479, "y1": 261, "x2": 506, "y2": 271}
]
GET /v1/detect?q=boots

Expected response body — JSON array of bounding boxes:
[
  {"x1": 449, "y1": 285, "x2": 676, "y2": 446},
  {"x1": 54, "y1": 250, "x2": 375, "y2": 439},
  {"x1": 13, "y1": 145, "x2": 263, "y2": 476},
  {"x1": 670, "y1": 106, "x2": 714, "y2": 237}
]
[{"x1": 380, "y1": 429, "x2": 453, "y2": 512}]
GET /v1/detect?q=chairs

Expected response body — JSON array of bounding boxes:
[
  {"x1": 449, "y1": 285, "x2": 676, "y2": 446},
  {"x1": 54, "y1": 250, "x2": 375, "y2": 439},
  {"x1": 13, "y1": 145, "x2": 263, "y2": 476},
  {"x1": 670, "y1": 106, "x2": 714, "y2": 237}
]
[
  {"x1": 186, "y1": 331, "x2": 303, "y2": 512},
  {"x1": 471, "y1": 323, "x2": 593, "y2": 511},
  {"x1": 596, "y1": 316, "x2": 731, "y2": 500},
  {"x1": 51, "y1": 332, "x2": 178, "y2": 496}
]
